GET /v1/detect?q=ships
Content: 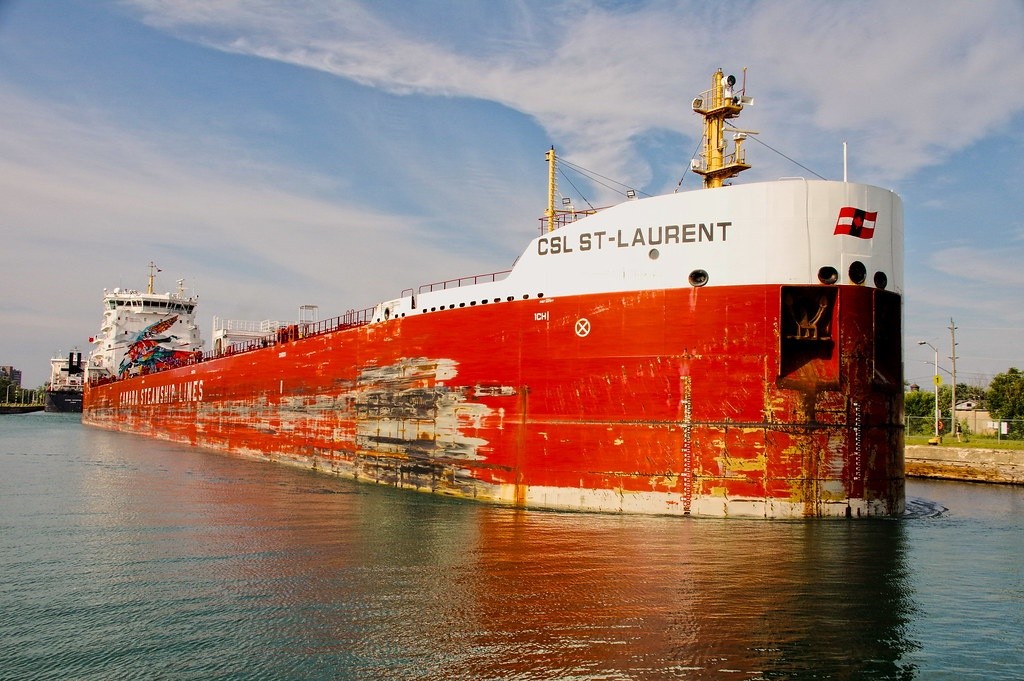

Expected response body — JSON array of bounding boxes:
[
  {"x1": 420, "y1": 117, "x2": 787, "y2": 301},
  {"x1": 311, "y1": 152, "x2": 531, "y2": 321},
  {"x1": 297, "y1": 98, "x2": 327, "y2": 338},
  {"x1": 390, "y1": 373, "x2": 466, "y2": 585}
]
[{"x1": 82, "y1": 69, "x2": 907, "y2": 521}]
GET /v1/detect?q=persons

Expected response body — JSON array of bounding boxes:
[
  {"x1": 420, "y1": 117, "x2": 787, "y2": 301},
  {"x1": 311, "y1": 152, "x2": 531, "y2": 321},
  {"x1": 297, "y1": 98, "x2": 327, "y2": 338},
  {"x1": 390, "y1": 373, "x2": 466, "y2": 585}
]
[
  {"x1": 956, "y1": 423, "x2": 962, "y2": 443},
  {"x1": 935, "y1": 418, "x2": 943, "y2": 444}
]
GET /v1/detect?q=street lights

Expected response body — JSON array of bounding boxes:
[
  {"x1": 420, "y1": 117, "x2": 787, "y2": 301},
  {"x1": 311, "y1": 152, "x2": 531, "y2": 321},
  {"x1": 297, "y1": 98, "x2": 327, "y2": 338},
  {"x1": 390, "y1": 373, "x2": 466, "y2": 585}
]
[
  {"x1": 6, "y1": 384, "x2": 15, "y2": 406},
  {"x1": 918, "y1": 341, "x2": 939, "y2": 437}
]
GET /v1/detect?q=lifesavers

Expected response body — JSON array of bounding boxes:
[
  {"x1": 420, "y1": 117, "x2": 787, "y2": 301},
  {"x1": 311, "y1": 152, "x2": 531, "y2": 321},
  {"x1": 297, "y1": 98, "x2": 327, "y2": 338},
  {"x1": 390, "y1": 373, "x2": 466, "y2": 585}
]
[
  {"x1": 287, "y1": 325, "x2": 298, "y2": 341},
  {"x1": 277, "y1": 329, "x2": 284, "y2": 344}
]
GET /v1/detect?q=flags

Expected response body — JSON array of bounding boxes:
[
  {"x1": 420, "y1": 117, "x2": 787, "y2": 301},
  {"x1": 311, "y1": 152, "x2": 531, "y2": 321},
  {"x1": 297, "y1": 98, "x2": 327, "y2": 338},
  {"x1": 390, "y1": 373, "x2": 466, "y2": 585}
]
[{"x1": 88, "y1": 336, "x2": 95, "y2": 343}]
